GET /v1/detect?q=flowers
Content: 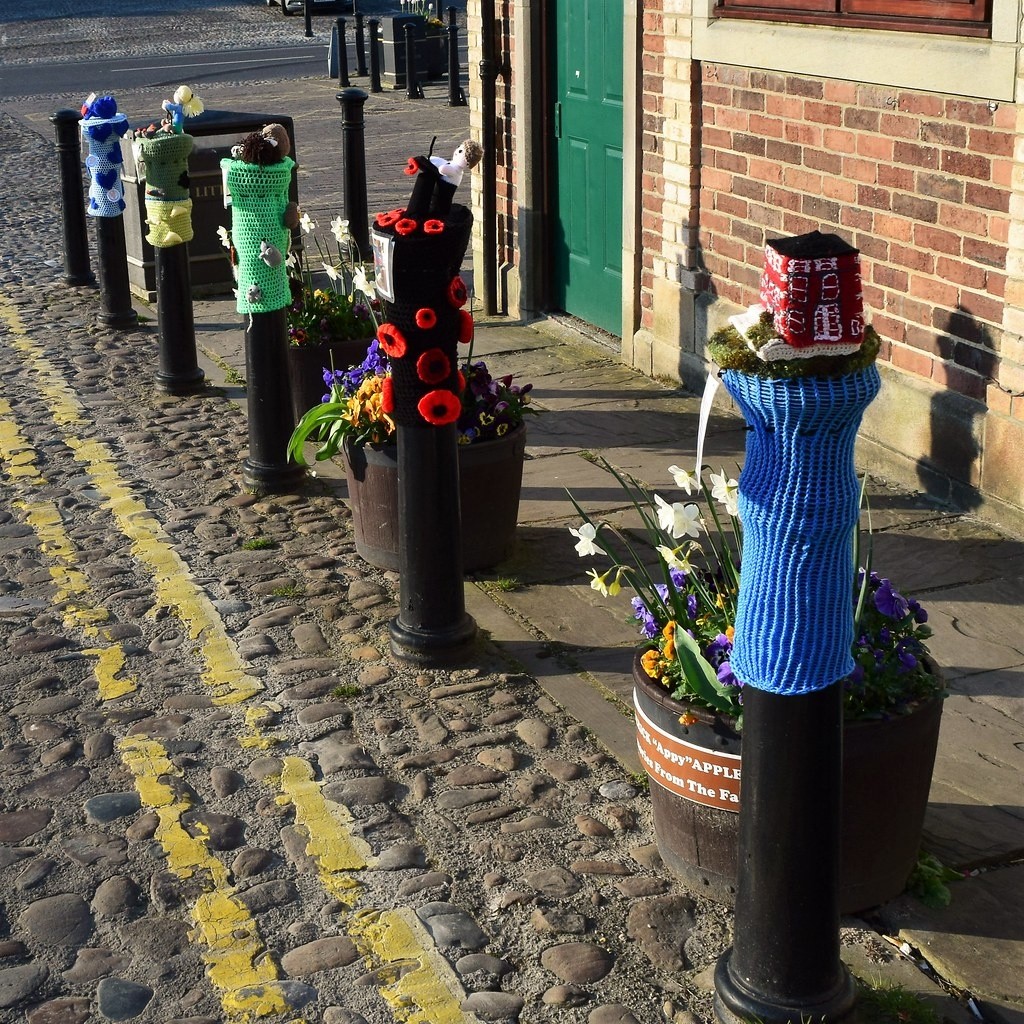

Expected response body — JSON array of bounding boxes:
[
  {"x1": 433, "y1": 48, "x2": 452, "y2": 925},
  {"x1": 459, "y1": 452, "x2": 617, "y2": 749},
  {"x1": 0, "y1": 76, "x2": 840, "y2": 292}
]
[
  {"x1": 218, "y1": 215, "x2": 385, "y2": 346},
  {"x1": 403, "y1": 158, "x2": 419, "y2": 177},
  {"x1": 449, "y1": 276, "x2": 467, "y2": 309},
  {"x1": 417, "y1": 348, "x2": 451, "y2": 383},
  {"x1": 381, "y1": 376, "x2": 394, "y2": 413},
  {"x1": 417, "y1": 389, "x2": 461, "y2": 425},
  {"x1": 286, "y1": 293, "x2": 537, "y2": 467},
  {"x1": 563, "y1": 456, "x2": 950, "y2": 727},
  {"x1": 377, "y1": 323, "x2": 406, "y2": 358},
  {"x1": 458, "y1": 370, "x2": 466, "y2": 393},
  {"x1": 424, "y1": 219, "x2": 444, "y2": 233},
  {"x1": 378, "y1": 208, "x2": 416, "y2": 236},
  {"x1": 400, "y1": 0, "x2": 446, "y2": 27},
  {"x1": 459, "y1": 309, "x2": 474, "y2": 344},
  {"x1": 416, "y1": 308, "x2": 437, "y2": 329}
]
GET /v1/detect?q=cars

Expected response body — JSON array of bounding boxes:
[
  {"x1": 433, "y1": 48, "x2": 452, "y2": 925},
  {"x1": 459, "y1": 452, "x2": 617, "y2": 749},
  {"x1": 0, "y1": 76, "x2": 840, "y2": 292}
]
[{"x1": 266, "y1": 0, "x2": 353, "y2": 15}]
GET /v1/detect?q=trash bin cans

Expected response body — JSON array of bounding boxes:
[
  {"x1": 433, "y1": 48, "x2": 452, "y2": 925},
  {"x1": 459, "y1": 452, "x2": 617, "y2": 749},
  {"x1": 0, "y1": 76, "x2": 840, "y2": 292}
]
[
  {"x1": 382, "y1": 13, "x2": 426, "y2": 85},
  {"x1": 118, "y1": 109, "x2": 305, "y2": 303}
]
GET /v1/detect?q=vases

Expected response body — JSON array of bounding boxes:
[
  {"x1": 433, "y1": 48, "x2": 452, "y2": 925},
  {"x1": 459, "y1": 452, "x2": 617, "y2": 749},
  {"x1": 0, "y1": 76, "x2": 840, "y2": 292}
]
[
  {"x1": 634, "y1": 650, "x2": 946, "y2": 912},
  {"x1": 287, "y1": 344, "x2": 368, "y2": 441},
  {"x1": 341, "y1": 423, "x2": 527, "y2": 572},
  {"x1": 425, "y1": 27, "x2": 449, "y2": 75}
]
